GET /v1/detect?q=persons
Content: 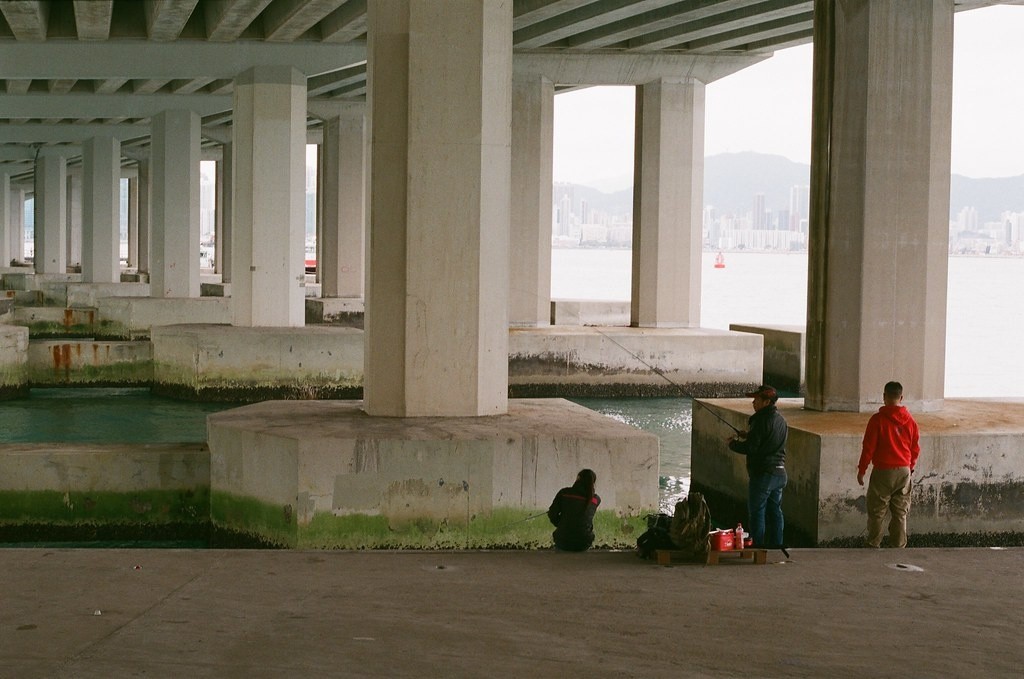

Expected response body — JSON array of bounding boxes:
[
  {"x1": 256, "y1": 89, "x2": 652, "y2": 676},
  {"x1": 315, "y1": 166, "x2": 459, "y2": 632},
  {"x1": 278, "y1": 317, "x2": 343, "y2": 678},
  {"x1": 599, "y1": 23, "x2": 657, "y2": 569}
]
[
  {"x1": 547, "y1": 469, "x2": 601, "y2": 552},
  {"x1": 725, "y1": 386, "x2": 787, "y2": 549},
  {"x1": 856, "y1": 382, "x2": 920, "y2": 548}
]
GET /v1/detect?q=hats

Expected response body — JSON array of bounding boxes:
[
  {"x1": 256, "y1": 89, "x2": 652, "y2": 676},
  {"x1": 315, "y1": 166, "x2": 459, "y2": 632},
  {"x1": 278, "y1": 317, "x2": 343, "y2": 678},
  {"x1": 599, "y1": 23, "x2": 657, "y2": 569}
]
[{"x1": 745, "y1": 388, "x2": 775, "y2": 398}]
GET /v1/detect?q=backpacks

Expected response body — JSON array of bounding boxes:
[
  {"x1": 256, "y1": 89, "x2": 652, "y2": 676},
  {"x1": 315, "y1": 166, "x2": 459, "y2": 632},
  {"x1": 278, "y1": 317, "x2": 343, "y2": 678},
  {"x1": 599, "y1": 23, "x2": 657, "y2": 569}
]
[{"x1": 669, "y1": 492, "x2": 712, "y2": 548}]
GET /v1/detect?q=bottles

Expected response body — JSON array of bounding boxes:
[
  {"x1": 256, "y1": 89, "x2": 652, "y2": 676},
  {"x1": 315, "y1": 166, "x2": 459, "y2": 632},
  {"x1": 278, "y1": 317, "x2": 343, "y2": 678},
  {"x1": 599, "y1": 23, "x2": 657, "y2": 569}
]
[{"x1": 735, "y1": 523, "x2": 744, "y2": 550}]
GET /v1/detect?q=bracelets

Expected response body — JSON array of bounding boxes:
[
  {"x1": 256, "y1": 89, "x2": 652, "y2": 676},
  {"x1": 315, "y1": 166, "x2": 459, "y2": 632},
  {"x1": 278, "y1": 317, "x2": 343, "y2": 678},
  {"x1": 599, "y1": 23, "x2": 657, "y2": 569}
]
[{"x1": 910, "y1": 470, "x2": 914, "y2": 474}]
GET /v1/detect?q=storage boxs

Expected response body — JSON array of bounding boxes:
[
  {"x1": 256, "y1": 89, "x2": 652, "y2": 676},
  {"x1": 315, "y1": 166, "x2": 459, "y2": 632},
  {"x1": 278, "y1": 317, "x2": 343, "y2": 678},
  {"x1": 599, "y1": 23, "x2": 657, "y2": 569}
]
[{"x1": 713, "y1": 531, "x2": 734, "y2": 550}]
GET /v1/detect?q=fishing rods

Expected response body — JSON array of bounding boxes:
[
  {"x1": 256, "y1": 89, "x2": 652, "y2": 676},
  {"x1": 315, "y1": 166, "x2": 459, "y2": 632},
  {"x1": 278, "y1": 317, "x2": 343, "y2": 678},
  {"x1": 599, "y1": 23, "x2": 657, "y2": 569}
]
[
  {"x1": 502, "y1": 510, "x2": 549, "y2": 530},
  {"x1": 859, "y1": 469, "x2": 915, "y2": 538},
  {"x1": 517, "y1": 285, "x2": 741, "y2": 441}
]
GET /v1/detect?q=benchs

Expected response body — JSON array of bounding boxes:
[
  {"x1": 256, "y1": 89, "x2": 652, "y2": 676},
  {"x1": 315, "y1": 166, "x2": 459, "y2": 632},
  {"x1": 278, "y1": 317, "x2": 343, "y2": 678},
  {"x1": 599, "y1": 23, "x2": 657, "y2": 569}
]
[{"x1": 655, "y1": 549, "x2": 767, "y2": 565}]
